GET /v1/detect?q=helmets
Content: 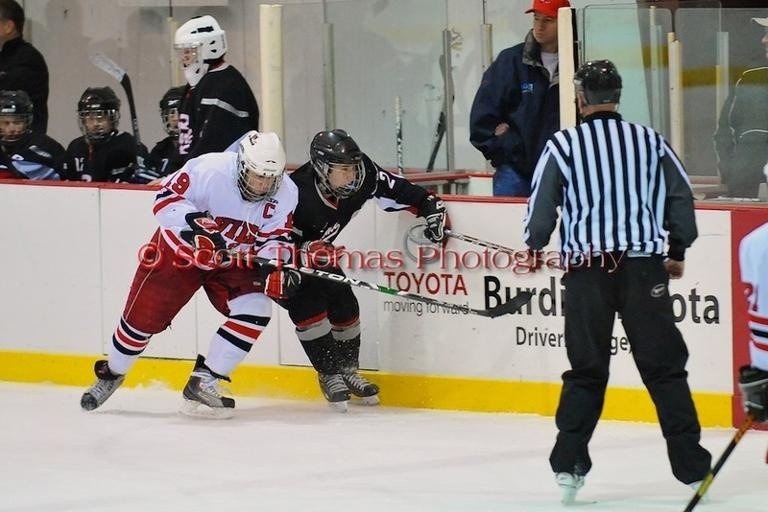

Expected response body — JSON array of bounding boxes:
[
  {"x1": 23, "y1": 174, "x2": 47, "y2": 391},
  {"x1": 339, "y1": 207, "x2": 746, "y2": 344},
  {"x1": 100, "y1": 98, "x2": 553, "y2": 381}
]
[
  {"x1": 157, "y1": 83, "x2": 191, "y2": 141},
  {"x1": 76, "y1": 86, "x2": 121, "y2": 142},
  {"x1": 236, "y1": 128, "x2": 289, "y2": 180},
  {"x1": 0, "y1": 88, "x2": 35, "y2": 151},
  {"x1": 307, "y1": 126, "x2": 364, "y2": 181},
  {"x1": 170, "y1": 13, "x2": 230, "y2": 88},
  {"x1": 571, "y1": 59, "x2": 623, "y2": 107}
]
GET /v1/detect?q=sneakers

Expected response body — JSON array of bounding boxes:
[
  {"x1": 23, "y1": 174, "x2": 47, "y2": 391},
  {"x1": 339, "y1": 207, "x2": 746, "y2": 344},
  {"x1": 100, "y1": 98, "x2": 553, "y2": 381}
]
[{"x1": 553, "y1": 471, "x2": 586, "y2": 489}]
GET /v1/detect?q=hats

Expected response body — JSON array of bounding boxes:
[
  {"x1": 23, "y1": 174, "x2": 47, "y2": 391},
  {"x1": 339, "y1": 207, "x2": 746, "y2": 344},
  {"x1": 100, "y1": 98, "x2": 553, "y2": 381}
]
[{"x1": 522, "y1": 0, "x2": 571, "y2": 19}]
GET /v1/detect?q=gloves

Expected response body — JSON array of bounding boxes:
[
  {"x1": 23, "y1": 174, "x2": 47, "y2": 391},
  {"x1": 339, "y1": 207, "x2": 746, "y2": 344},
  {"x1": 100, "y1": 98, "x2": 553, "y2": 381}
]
[
  {"x1": 177, "y1": 208, "x2": 229, "y2": 264},
  {"x1": 300, "y1": 238, "x2": 346, "y2": 271},
  {"x1": 737, "y1": 363, "x2": 768, "y2": 425},
  {"x1": 420, "y1": 192, "x2": 450, "y2": 245},
  {"x1": 252, "y1": 235, "x2": 309, "y2": 297}
]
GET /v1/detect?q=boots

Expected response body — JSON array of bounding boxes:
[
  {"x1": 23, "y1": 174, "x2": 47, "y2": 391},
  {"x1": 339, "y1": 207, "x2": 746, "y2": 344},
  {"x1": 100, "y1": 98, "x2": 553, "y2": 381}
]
[
  {"x1": 340, "y1": 360, "x2": 384, "y2": 397},
  {"x1": 80, "y1": 358, "x2": 127, "y2": 411},
  {"x1": 316, "y1": 367, "x2": 353, "y2": 404},
  {"x1": 180, "y1": 353, "x2": 237, "y2": 410}
]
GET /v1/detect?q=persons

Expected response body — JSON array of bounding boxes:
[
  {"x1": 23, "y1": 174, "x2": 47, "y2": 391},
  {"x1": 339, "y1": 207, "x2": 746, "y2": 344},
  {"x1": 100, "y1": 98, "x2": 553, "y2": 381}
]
[
  {"x1": 81, "y1": 130, "x2": 299, "y2": 411},
  {"x1": 271, "y1": 129, "x2": 448, "y2": 402},
  {"x1": 523, "y1": 60, "x2": 712, "y2": 485},
  {"x1": 737, "y1": 222, "x2": 768, "y2": 465},
  {"x1": 469, "y1": 0, "x2": 582, "y2": 196},
  {"x1": 713, "y1": 17, "x2": 768, "y2": 199}
]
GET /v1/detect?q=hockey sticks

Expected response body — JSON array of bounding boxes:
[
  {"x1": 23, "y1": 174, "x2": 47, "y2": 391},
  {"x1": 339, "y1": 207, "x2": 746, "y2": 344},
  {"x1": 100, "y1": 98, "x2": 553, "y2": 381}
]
[
  {"x1": 227, "y1": 250, "x2": 532, "y2": 316},
  {"x1": 427, "y1": 55, "x2": 455, "y2": 172},
  {"x1": 90, "y1": 49, "x2": 146, "y2": 185}
]
